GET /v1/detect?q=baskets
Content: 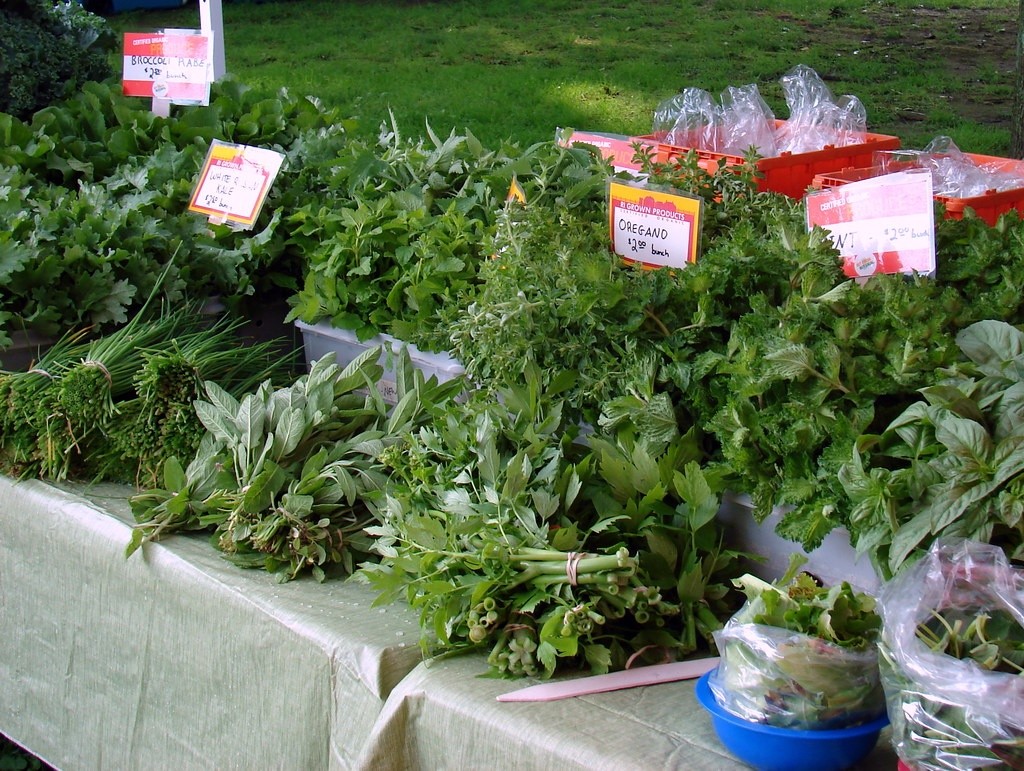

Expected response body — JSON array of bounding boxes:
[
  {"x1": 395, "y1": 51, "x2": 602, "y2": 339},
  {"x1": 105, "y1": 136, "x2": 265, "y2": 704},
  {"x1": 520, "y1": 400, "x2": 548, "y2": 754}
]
[
  {"x1": 630, "y1": 118, "x2": 901, "y2": 206},
  {"x1": 812, "y1": 151, "x2": 1024, "y2": 228}
]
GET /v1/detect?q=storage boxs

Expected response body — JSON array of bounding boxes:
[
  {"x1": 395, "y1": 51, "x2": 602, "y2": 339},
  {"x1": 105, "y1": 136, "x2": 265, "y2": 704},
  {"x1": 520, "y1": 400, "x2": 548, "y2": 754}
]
[
  {"x1": 631, "y1": 118, "x2": 900, "y2": 204},
  {"x1": 812, "y1": 152, "x2": 1024, "y2": 235}
]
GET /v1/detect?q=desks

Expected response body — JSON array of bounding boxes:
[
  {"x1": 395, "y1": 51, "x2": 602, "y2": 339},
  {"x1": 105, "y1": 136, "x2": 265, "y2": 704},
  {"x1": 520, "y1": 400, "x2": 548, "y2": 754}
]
[
  {"x1": 357, "y1": 653, "x2": 899, "y2": 771},
  {"x1": 0, "y1": 469, "x2": 427, "y2": 771}
]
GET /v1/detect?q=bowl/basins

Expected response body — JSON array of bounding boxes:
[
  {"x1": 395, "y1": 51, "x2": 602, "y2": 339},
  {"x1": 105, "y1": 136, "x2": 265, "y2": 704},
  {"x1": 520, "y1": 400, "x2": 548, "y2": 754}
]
[{"x1": 695, "y1": 668, "x2": 890, "y2": 771}]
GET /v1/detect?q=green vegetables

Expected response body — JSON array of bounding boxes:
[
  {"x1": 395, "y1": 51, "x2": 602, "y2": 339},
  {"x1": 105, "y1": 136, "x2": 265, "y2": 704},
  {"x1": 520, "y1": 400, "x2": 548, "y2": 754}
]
[{"x1": 0, "y1": 0, "x2": 1024, "y2": 771}]
